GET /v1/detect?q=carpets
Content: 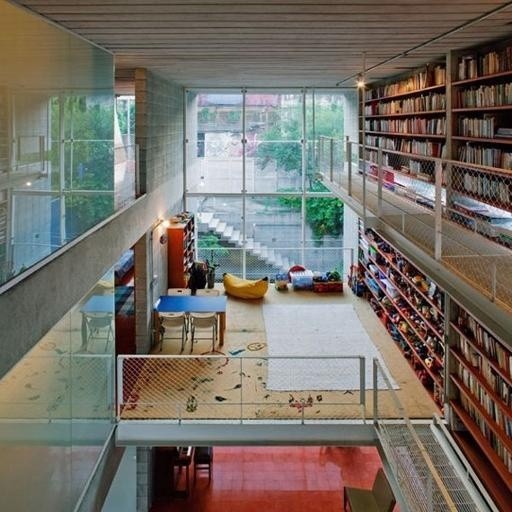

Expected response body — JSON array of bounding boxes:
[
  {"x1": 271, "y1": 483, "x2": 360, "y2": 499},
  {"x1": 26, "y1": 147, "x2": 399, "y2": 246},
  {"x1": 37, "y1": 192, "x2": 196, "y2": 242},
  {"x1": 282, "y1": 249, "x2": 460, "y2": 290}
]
[{"x1": 262, "y1": 304, "x2": 400, "y2": 390}]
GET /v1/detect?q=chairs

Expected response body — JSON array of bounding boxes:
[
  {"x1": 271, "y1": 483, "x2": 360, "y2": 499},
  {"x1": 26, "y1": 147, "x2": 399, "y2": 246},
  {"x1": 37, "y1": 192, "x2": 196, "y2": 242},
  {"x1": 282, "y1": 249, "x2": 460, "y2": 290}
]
[
  {"x1": 158, "y1": 288, "x2": 220, "y2": 352},
  {"x1": 84, "y1": 315, "x2": 114, "y2": 352},
  {"x1": 344, "y1": 469, "x2": 396, "y2": 512}
]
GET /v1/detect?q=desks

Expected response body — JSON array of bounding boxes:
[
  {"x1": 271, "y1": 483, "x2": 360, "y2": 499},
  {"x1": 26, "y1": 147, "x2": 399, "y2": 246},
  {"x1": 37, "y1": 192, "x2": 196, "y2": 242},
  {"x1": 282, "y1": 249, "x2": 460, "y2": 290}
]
[
  {"x1": 154, "y1": 296, "x2": 227, "y2": 346},
  {"x1": 79, "y1": 294, "x2": 115, "y2": 348}
]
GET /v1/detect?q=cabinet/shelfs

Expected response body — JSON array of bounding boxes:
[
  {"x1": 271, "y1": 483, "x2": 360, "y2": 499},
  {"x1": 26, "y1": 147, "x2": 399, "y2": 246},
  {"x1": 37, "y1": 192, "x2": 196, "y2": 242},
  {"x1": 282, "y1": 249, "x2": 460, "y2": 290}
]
[
  {"x1": 359, "y1": 36, "x2": 511, "y2": 247},
  {"x1": 437, "y1": 294, "x2": 512, "y2": 512},
  {"x1": 168, "y1": 212, "x2": 195, "y2": 288},
  {"x1": 358, "y1": 221, "x2": 445, "y2": 412}
]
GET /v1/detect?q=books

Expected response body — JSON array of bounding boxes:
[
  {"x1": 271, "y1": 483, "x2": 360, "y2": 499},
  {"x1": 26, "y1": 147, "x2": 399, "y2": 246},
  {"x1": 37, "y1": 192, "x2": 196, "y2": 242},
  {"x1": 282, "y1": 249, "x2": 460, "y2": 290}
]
[{"x1": 359, "y1": 45, "x2": 512, "y2": 212}]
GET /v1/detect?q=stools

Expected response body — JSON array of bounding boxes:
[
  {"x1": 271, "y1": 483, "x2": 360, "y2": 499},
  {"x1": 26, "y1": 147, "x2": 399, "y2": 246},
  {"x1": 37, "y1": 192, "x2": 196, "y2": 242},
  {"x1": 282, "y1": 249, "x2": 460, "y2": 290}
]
[{"x1": 193, "y1": 446, "x2": 213, "y2": 486}]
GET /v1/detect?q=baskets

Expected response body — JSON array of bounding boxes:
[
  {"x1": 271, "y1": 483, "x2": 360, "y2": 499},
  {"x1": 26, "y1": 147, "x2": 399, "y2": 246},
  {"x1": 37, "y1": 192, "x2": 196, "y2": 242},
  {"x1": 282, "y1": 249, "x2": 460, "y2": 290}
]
[{"x1": 290, "y1": 269, "x2": 313, "y2": 289}]
